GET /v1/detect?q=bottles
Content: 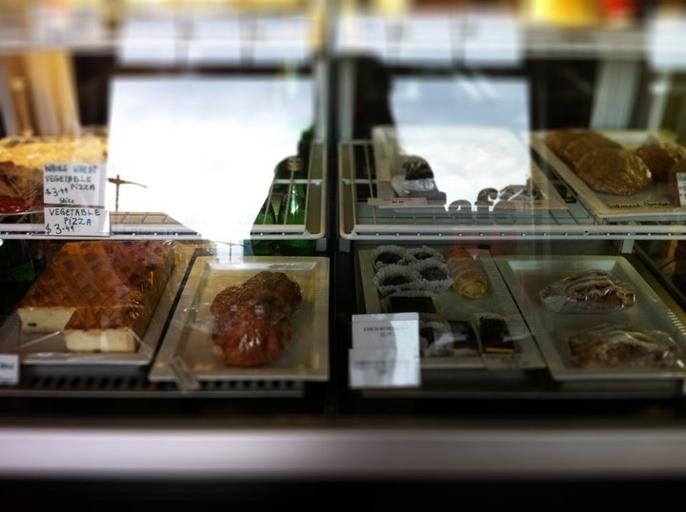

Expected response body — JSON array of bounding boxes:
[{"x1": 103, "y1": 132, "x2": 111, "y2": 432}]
[{"x1": 250, "y1": 156, "x2": 308, "y2": 257}]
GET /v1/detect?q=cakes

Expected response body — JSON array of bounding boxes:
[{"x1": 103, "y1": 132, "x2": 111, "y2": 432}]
[{"x1": 370, "y1": 244, "x2": 520, "y2": 358}]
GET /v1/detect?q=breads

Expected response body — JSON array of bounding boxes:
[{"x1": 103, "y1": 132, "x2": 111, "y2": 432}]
[
  {"x1": 545, "y1": 122, "x2": 686, "y2": 206},
  {"x1": 16, "y1": 239, "x2": 176, "y2": 351},
  {"x1": 1, "y1": 159, "x2": 44, "y2": 223},
  {"x1": 537, "y1": 270, "x2": 637, "y2": 311},
  {"x1": 207, "y1": 269, "x2": 304, "y2": 369},
  {"x1": 567, "y1": 327, "x2": 683, "y2": 370}
]
